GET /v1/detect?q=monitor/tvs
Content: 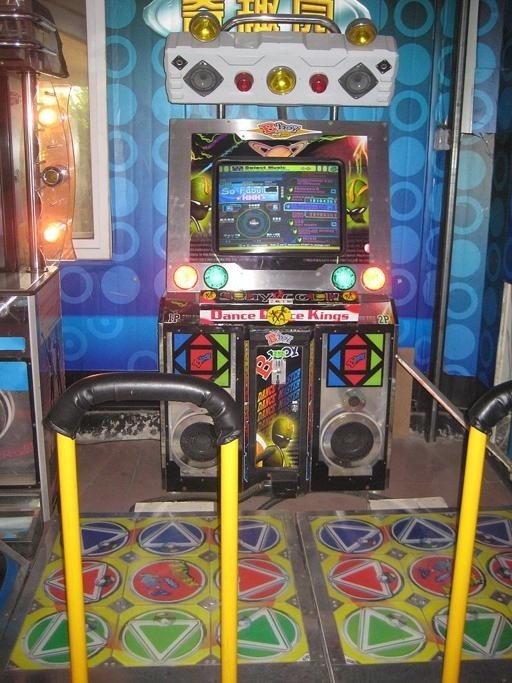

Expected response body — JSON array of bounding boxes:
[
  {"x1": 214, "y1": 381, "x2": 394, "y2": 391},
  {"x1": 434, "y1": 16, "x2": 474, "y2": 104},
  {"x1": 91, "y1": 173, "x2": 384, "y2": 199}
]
[{"x1": 212, "y1": 158, "x2": 344, "y2": 256}]
